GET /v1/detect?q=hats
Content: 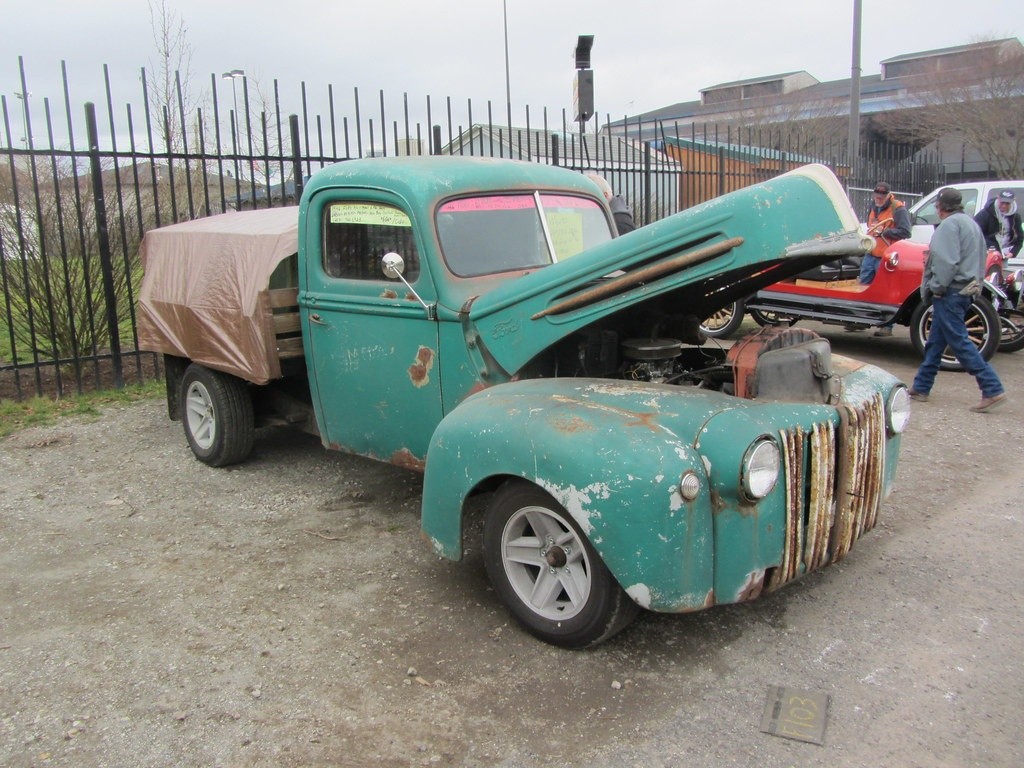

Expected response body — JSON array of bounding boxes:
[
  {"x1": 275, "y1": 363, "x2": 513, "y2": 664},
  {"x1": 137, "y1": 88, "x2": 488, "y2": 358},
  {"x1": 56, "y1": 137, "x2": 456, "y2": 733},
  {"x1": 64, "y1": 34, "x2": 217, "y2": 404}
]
[
  {"x1": 999, "y1": 189, "x2": 1015, "y2": 204},
  {"x1": 874, "y1": 182, "x2": 892, "y2": 194},
  {"x1": 935, "y1": 188, "x2": 964, "y2": 211}
]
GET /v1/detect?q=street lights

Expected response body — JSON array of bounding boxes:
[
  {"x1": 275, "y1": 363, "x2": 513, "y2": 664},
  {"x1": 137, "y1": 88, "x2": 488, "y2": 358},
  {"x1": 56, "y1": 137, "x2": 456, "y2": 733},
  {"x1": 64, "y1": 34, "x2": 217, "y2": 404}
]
[{"x1": 223, "y1": 70, "x2": 245, "y2": 181}]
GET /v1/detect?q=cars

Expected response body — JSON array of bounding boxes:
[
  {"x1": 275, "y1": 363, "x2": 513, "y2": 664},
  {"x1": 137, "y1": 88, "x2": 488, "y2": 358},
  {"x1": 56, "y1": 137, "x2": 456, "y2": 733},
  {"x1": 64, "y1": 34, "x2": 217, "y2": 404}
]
[
  {"x1": 700, "y1": 217, "x2": 1023, "y2": 374},
  {"x1": 905, "y1": 181, "x2": 1024, "y2": 293}
]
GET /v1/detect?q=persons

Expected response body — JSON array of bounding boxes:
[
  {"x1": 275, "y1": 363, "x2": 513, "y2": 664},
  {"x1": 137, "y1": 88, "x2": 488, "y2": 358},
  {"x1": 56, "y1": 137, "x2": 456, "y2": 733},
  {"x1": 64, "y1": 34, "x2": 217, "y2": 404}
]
[
  {"x1": 908, "y1": 185, "x2": 1008, "y2": 416},
  {"x1": 971, "y1": 188, "x2": 1024, "y2": 332},
  {"x1": 843, "y1": 183, "x2": 910, "y2": 336},
  {"x1": 588, "y1": 173, "x2": 635, "y2": 239}
]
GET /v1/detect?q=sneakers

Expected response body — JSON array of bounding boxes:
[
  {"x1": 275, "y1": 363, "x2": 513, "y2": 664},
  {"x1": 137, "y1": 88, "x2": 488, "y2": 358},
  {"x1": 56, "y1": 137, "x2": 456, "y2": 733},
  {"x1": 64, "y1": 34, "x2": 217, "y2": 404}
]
[
  {"x1": 977, "y1": 393, "x2": 1007, "y2": 413},
  {"x1": 908, "y1": 389, "x2": 928, "y2": 402},
  {"x1": 876, "y1": 326, "x2": 894, "y2": 337}
]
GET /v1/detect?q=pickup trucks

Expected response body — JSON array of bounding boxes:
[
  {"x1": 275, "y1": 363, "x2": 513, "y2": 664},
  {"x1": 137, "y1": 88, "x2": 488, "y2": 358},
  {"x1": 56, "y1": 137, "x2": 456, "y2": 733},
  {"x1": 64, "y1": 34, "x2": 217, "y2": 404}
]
[{"x1": 140, "y1": 154, "x2": 913, "y2": 648}]
